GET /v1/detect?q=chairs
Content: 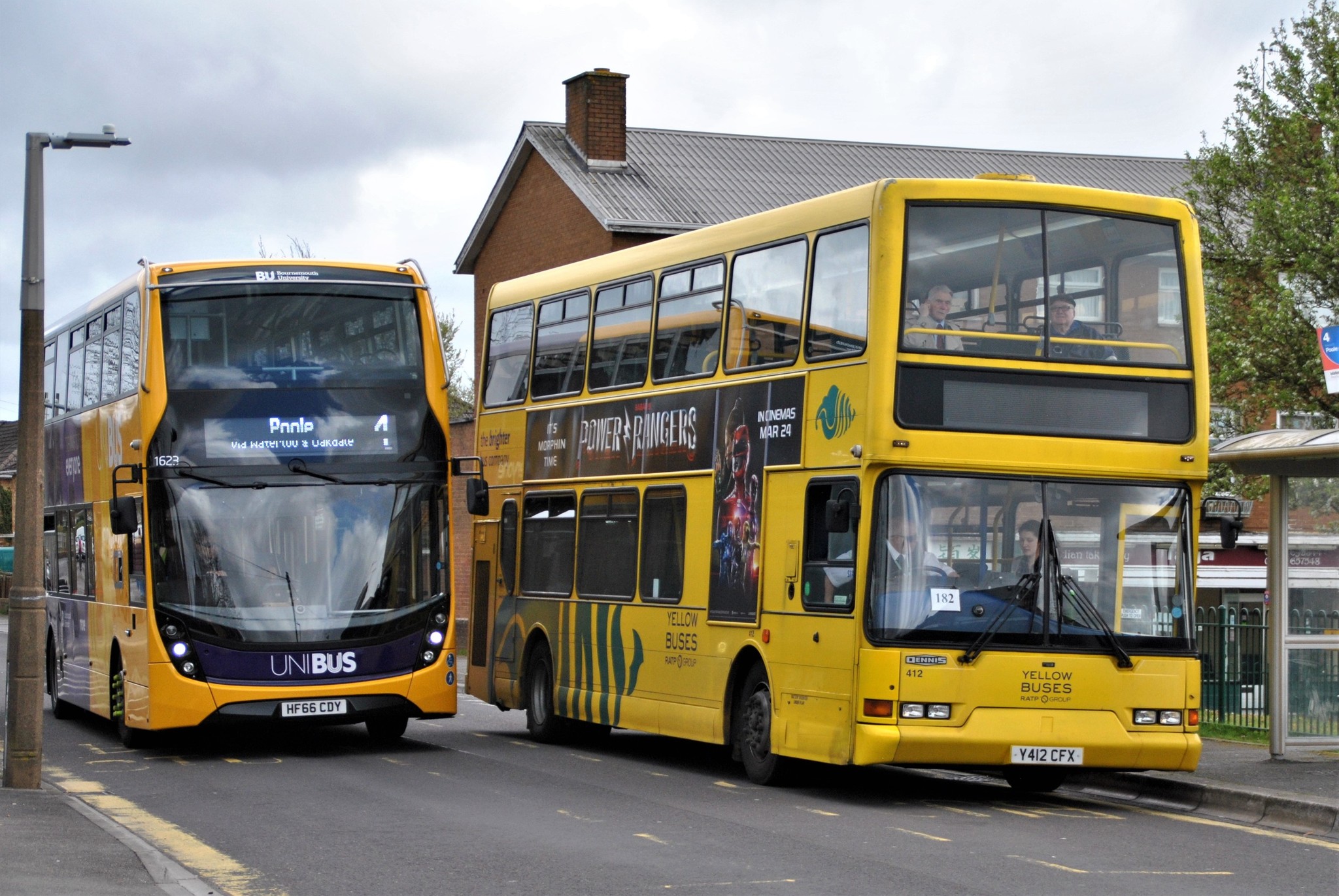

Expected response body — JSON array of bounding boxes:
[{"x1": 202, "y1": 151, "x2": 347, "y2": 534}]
[{"x1": 324, "y1": 345, "x2": 412, "y2": 381}]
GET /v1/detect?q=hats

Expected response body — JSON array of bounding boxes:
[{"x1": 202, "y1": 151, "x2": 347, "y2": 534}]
[{"x1": 1050, "y1": 294, "x2": 1076, "y2": 307}]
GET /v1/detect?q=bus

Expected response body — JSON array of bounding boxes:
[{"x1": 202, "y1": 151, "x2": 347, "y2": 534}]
[
  {"x1": 471, "y1": 172, "x2": 1246, "y2": 800},
  {"x1": 41, "y1": 256, "x2": 492, "y2": 752}
]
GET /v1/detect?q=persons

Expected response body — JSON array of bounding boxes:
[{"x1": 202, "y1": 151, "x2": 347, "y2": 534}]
[
  {"x1": 823, "y1": 519, "x2": 977, "y2": 604},
  {"x1": 1028, "y1": 293, "x2": 1118, "y2": 361},
  {"x1": 1010, "y1": 519, "x2": 1064, "y2": 624},
  {"x1": 904, "y1": 285, "x2": 965, "y2": 350},
  {"x1": 714, "y1": 398, "x2": 760, "y2": 616},
  {"x1": 171, "y1": 531, "x2": 240, "y2": 607}
]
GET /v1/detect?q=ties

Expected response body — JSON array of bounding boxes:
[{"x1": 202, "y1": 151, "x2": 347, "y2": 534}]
[
  {"x1": 935, "y1": 325, "x2": 944, "y2": 350},
  {"x1": 896, "y1": 555, "x2": 905, "y2": 569}
]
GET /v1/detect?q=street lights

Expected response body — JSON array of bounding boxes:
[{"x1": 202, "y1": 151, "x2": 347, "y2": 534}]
[{"x1": 0, "y1": 121, "x2": 132, "y2": 792}]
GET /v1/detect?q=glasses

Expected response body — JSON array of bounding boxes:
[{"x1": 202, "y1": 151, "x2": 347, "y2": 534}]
[
  {"x1": 892, "y1": 535, "x2": 918, "y2": 542},
  {"x1": 1050, "y1": 305, "x2": 1073, "y2": 312},
  {"x1": 195, "y1": 541, "x2": 222, "y2": 548}
]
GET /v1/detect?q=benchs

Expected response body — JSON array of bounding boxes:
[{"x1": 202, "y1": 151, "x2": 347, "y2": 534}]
[{"x1": 961, "y1": 316, "x2": 1132, "y2": 358}]
